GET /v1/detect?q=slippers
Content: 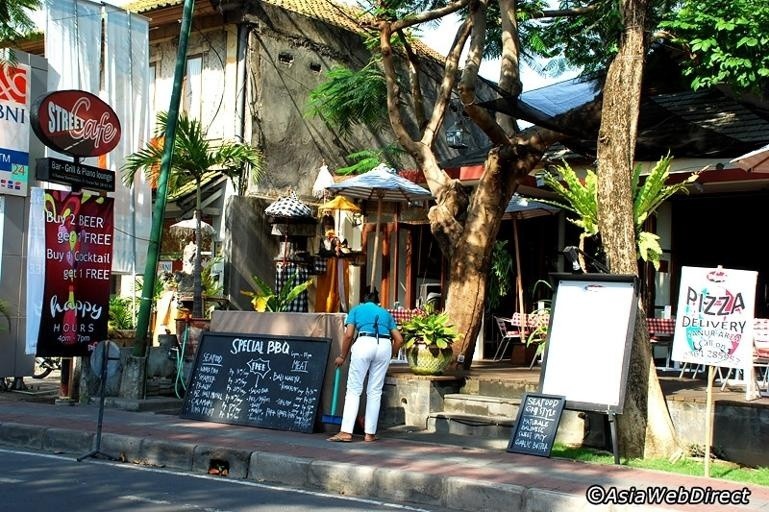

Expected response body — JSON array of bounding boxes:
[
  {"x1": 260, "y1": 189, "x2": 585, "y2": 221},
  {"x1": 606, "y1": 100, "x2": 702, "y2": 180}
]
[
  {"x1": 326, "y1": 433, "x2": 353, "y2": 442},
  {"x1": 363, "y1": 434, "x2": 380, "y2": 442}
]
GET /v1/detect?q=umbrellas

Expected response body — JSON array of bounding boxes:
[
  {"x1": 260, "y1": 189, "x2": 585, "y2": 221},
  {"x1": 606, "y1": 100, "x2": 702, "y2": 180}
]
[
  {"x1": 169, "y1": 210, "x2": 219, "y2": 246},
  {"x1": 318, "y1": 195, "x2": 362, "y2": 245},
  {"x1": 311, "y1": 158, "x2": 336, "y2": 203},
  {"x1": 328, "y1": 162, "x2": 437, "y2": 294},
  {"x1": 500, "y1": 191, "x2": 574, "y2": 341},
  {"x1": 261, "y1": 188, "x2": 315, "y2": 275}
]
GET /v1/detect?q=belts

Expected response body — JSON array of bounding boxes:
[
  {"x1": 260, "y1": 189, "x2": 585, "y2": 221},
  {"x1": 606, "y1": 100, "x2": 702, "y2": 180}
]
[{"x1": 359, "y1": 332, "x2": 390, "y2": 339}]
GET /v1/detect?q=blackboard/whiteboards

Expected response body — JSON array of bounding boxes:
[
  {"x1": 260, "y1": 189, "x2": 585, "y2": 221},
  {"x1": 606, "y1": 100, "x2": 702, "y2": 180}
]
[
  {"x1": 506, "y1": 392, "x2": 565, "y2": 457},
  {"x1": 179, "y1": 331, "x2": 333, "y2": 434},
  {"x1": 538, "y1": 272, "x2": 640, "y2": 415}
]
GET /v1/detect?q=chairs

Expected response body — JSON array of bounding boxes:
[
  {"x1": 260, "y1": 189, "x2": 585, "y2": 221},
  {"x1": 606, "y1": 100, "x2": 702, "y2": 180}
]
[
  {"x1": 645, "y1": 315, "x2": 769, "y2": 396},
  {"x1": 492, "y1": 313, "x2": 549, "y2": 364}
]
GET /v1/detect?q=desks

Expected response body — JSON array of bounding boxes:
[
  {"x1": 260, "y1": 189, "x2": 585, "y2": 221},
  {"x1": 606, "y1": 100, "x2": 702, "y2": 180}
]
[{"x1": 388, "y1": 309, "x2": 423, "y2": 324}]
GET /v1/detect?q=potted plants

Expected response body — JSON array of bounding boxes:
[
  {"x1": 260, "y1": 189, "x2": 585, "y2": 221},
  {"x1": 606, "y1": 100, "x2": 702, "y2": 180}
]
[
  {"x1": 397, "y1": 312, "x2": 461, "y2": 375},
  {"x1": 107, "y1": 295, "x2": 137, "y2": 348}
]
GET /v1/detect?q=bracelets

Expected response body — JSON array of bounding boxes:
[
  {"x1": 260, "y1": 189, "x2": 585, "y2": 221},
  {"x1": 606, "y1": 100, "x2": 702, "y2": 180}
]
[{"x1": 338, "y1": 353, "x2": 346, "y2": 359}]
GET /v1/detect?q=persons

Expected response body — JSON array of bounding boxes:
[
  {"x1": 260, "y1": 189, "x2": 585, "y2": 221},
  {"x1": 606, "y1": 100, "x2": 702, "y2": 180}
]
[{"x1": 323, "y1": 292, "x2": 403, "y2": 442}]
